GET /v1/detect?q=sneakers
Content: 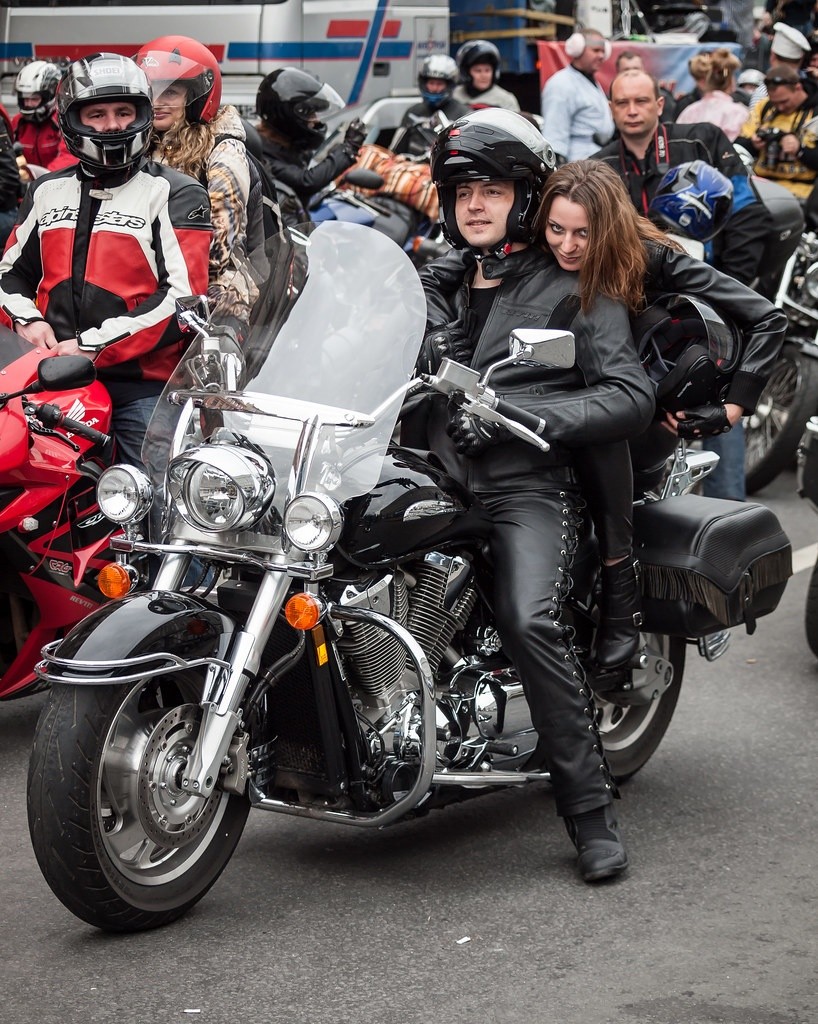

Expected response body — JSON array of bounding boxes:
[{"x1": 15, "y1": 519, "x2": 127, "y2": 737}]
[{"x1": 566, "y1": 802, "x2": 628, "y2": 882}]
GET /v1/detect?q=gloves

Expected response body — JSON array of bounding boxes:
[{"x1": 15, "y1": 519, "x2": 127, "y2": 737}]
[
  {"x1": 415, "y1": 327, "x2": 471, "y2": 379},
  {"x1": 445, "y1": 404, "x2": 505, "y2": 456},
  {"x1": 343, "y1": 114, "x2": 367, "y2": 160}
]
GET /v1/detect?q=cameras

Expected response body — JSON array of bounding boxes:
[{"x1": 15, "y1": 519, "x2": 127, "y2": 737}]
[{"x1": 757, "y1": 127, "x2": 788, "y2": 171}]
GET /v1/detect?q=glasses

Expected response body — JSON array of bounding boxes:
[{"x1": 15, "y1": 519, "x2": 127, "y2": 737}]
[{"x1": 764, "y1": 78, "x2": 795, "y2": 87}]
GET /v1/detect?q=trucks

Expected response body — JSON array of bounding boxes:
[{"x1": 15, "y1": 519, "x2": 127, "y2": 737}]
[
  {"x1": 22, "y1": 222, "x2": 794, "y2": 926},
  {"x1": 2, "y1": 2, "x2": 743, "y2": 167}
]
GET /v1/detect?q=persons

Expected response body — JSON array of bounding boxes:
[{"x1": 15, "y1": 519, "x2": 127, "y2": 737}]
[
  {"x1": 754, "y1": 0, "x2": 818, "y2": 48},
  {"x1": 452, "y1": 40, "x2": 520, "y2": 113},
  {"x1": 616, "y1": 51, "x2": 676, "y2": 122},
  {"x1": 391, "y1": 54, "x2": 471, "y2": 163},
  {"x1": 674, "y1": 51, "x2": 753, "y2": 115},
  {"x1": 1, "y1": 60, "x2": 81, "y2": 248},
  {"x1": 736, "y1": 70, "x2": 766, "y2": 91},
  {"x1": 770, "y1": 22, "x2": 811, "y2": 82},
  {"x1": 256, "y1": 66, "x2": 369, "y2": 197},
  {"x1": 542, "y1": 30, "x2": 615, "y2": 164},
  {"x1": 803, "y1": 43, "x2": 818, "y2": 79},
  {"x1": 585, "y1": 70, "x2": 773, "y2": 502},
  {"x1": 733, "y1": 64, "x2": 818, "y2": 206},
  {"x1": 400, "y1": 106, "x2": 789, "y2": 880},
  {"x1": 129, "y1": 35, "x2": 260, "y2": 438},
  {"x1": 1, "y1": 52, "x2": 211, "y2": 564},
  {"x1": 677, "y1": 48, "x2": 751, "y2": 144}
]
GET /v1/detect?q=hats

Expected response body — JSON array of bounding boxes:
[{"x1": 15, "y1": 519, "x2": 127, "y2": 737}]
[
  {"x1": 738, "y1": 68, "x2": 766, "y2": 86},
  {"x1": 771, "y1": 23, "x2": 812, "y2": 59}
]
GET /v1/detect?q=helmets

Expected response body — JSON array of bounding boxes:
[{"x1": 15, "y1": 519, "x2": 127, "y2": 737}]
[
  {"x1": 456, "y1": 40, "x2": 502, "y2": 86},
  {"x1": 647, "y1": 159, "x2": 733, "y2": 245},
  {"x1": 417, "y1": 53, "x2": 459, "y2": 108},
  {"x1": 137, "y1": 35, "x2": 223, "y2": 125},
  {"x1": 417, "y1": 104, "x2": 556, "y2": 251},
  {"x1": 15, "y1": 61, "x2": 63, "y2": 122},
  {"x1": 55, "y1": 52, "x2": 153, "y2": 169},
  {"x1": 643, "y1": 295, "x2": 742, "y2": 413},
  {"x1": 255, "y1": 67, "x2": 328, "y2": 152}
]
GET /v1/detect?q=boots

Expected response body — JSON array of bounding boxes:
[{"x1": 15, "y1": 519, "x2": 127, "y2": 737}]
[{"x1": 597, "y1": 554, "x2": 644, "y2": 670}]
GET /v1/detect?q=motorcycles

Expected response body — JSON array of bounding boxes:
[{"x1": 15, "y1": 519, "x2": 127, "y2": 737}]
[
  {"x1": 0, "y1": 331, "x2": 146, "y2": 701},
  {"x1": 294, "y1": 169, "x2": 446, "y2": 252},
  {"x1": 649, "y1": 215, "x2": 818, "y2": 664}
]
